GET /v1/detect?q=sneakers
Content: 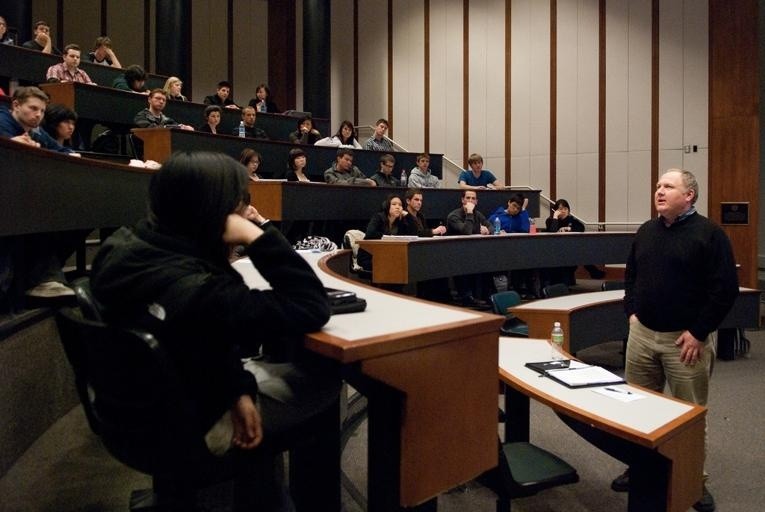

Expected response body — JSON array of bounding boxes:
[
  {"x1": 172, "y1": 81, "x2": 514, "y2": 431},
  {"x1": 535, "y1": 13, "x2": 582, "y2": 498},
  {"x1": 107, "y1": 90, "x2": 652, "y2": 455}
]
[
  {"x1": 26, "y1": 282, "x2": 75, "y2": 302},
  {"x1": 693, "y1": 486, "x2": 715, "y2": 510},
  {"x1": 611, "y1": 468, "x2": 631, "y2": 492}
]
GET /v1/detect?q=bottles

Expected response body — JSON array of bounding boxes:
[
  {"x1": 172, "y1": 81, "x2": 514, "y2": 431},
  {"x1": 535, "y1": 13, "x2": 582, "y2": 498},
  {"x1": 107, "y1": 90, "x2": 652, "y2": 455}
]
[
  {"x1": 530, "y1": 218, "x2": 536, "y2": 233},
  {"x1": 494, "y1": 216, "x2": 501, "y2": 234},
  {"x1": 549, "y1": 321, "x2": 563, "y2": 363}
]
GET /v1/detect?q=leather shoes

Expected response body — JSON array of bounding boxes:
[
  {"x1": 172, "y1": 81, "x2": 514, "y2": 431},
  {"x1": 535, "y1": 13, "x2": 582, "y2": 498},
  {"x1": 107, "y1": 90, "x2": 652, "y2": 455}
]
[{"x1": 462, "y1": 295, "x2": 486, "y2": 306}]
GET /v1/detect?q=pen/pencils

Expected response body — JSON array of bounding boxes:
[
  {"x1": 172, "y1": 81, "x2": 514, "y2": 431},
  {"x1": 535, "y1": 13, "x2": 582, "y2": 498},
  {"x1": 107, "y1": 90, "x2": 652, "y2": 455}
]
[{"x1": 605, "y1": 388, "x2": 631, "y2": 394}]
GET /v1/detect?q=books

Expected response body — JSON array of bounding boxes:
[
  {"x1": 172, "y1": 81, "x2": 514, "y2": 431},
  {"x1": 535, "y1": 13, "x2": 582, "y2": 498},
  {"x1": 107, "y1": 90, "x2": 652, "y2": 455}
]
[{"x1": 525, "y1": 357, "x2": 626, "y2": 390}]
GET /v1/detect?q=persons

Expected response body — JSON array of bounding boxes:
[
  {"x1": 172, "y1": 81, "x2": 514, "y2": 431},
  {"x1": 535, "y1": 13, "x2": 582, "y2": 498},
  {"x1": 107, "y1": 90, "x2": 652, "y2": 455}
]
[
  {"x1": 66, "y1": 152, "x2": 346, "y2": 512},
  {"x1": 135, "y1": 87, "x2": 500, "y2": 188},
  {"x1": 0, "y1": 16, "x2": 279, "y2": 297},
  {"x1": 357, "y1": 189, "x2": 584, "y2": 304},
  {"x1": 610, "y1": 166, "x2": 740, "y2": 512}
]
